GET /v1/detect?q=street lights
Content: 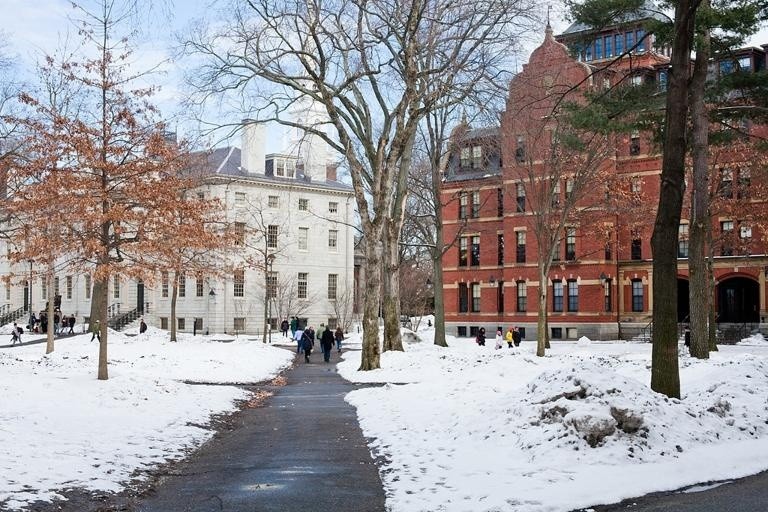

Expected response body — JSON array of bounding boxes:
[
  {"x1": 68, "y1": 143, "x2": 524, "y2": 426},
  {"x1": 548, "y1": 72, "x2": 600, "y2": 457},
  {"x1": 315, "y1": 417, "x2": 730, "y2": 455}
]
[
  {"x1": 26, "y1": 257, "x2": 37, "y2": 315},
  {"x1": 266, "y1": 252, "x2": 274, "y2": 344}
]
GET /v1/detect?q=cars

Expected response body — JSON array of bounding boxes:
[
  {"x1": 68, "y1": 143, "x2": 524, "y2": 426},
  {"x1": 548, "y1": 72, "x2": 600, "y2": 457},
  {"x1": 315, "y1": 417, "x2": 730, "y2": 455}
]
[{"x1": 399, "y1": 314, "x2": 412, "y2": 323}]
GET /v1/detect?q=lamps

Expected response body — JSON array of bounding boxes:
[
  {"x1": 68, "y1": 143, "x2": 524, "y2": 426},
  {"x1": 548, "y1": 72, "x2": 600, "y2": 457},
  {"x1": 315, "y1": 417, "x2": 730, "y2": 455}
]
[
  {"x1": 599, "y1": 271, "x2": 613, "y2": 312},
  {"x1": 489, "y1": 275, "x2": 504, "y2": 313}
]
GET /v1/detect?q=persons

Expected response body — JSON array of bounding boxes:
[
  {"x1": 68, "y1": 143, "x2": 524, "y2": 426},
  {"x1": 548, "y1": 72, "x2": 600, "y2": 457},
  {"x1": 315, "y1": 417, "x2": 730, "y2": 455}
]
[
  {"x1": 495, "y1": 326, "x2": 503, "y2": 349},
  {"x1": 90, "y1": 320, "x2": 101, "y2": 342},
  {"x1": 308, "y1": 326, "x2": 315, "y2": 345},
  {"x1": 11, "y1": 322, "x2": 23, "y2": 346},
  {"x1": 315, "y1": 322, "x2": 326, "y2": 353},
  {"x1": 280, "y1": 316, "x2": 289, "y2": 337},
  {"x1": 320, "y1": 324, "x2": 336, "y2": 362},
  {"x1": 26, "y1": 291, "x2": 77, "y2": 336},
  {"x1": 513, "y1": 325, "x2": 522, "y2": 347},
  {"x1": 300, "y1": 328, "x2": 314, "y2": 364},
  {"x1": 289, "y1": 316, "x2": 297, "y2": 339},
  {"x1": 505, "y1": 326, "x2": 514, "y2": 348},
  {"x1": 139, "y1": 318, "x2": 148, "y2": 334},
  {"x1": 477, "y1": 326, "x2": 486, "y2": 346},
  {"x1": 335, "y1": 327, "x2": 345, "y2": 352},
  {"x1": 293, "y1": 326, "x2": 304, "y2": 353}
]
